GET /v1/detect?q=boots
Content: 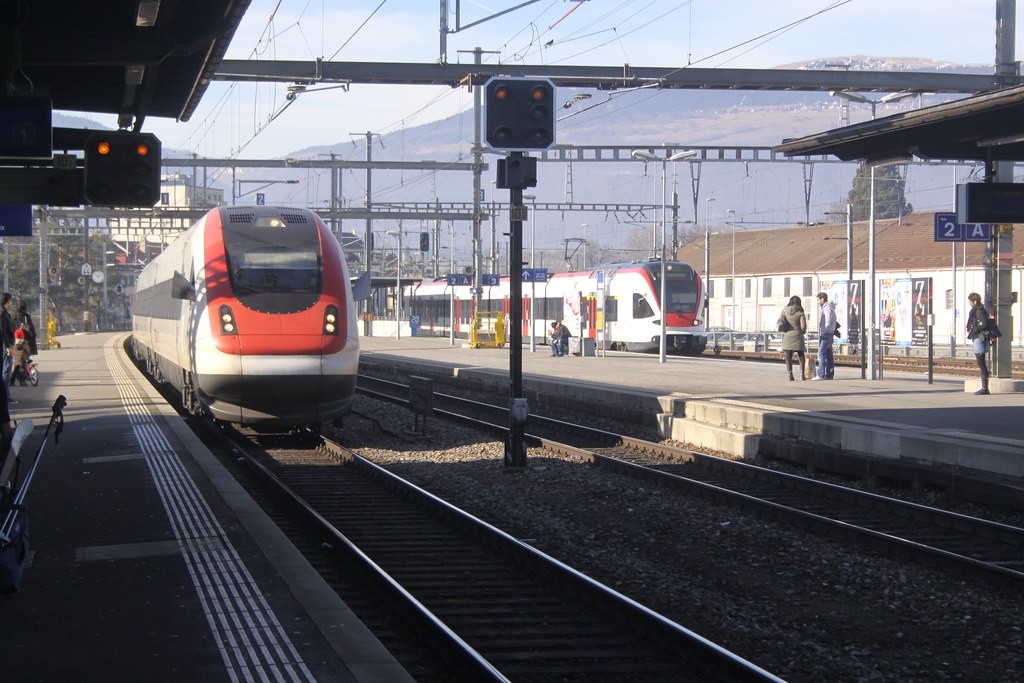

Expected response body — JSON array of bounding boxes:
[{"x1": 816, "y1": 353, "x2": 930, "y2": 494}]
[
  {"x1": 786, "y1": 363, "x2": 795, "y2": 381},
  {"x1": 800, "y1": 361, "x2": 807, "y2": 380}
]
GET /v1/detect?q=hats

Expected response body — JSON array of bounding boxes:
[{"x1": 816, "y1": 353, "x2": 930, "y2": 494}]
[{"x1": 14, "y1": 327, "x2": 25, "y2": 339}]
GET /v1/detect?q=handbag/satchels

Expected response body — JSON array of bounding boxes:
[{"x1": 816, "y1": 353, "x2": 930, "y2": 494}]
[
  {"x1": 779, "y1": 308, "x2": 789, "y2": 332},
  {"x1": 21, "y1": 315, "x2": 30, "y2": 332},
  {"x1": 977, "y1": 307, "x2": 1002, "y2": 343}
]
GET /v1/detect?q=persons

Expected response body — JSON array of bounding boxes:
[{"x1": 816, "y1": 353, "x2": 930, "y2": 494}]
[
  {"x1": 966, "y1": 292, "x2": 990, "y2": 395},
  {"x1": 811, "y1": 292, "x2": 838, "y2": 380},
  {"x1": 776, "y1": 295, "x2": 807, "y2": 381},
  {"x1": 547, "y1": 322, "x2": 572, "y2": 357},
  {"x1": 0, "y1": 292, "x2": 37, "y2": 422}
]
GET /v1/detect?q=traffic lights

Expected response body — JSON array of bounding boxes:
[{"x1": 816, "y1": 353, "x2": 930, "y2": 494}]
[
  {"x1": 485, "y1": 77, "x2": 557, "y2": 151},
  {"x1": 84, "y1": 133, "x2": 162, "y2": 208},
  {"x1": 420, "y1": 232, "x2": 429, "y2": 251}
]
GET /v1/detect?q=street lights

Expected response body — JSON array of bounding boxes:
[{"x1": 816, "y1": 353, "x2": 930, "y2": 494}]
[
  {"x1": 581, "y1": 222, "x2": 589, "y2": 271},
  {"x1": 727, "y1": 210, "x2": 735, "y2": 330},
  {"x1": 523, "y1": 194, "x2": 536, "y2": 352},
  {"x1": 830, "y1": 91, "x2": 918, "y2": 381},
  {"x1": 632, "y1": 150, "x2": 698, "y2": 364},
  {"x1": 491, "y1": 181, "x2": 497, "y2": 276},
  {"x1": 823, "y1": 203, "x2": 853, "y2": 358},
  {"x1": 704, "y1": 198, "x2": 716, "y2": 333}
]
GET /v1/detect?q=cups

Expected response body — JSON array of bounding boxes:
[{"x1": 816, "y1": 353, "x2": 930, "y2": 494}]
[{"x1": 548, "y1": 330, "x2": 552, "y2": 334}]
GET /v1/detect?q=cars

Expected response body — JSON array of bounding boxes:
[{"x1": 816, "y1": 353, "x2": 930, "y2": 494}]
[{"x1": 705, "y1": 329, "x2": 819, "y2": 352}]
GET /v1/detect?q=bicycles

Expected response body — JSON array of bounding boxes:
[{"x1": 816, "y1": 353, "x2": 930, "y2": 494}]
[{"x1": 25, "y1": 359, "x2": 39, "y2": 387}]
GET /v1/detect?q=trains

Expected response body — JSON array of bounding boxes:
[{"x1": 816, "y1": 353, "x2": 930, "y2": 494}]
[
  {"x1": 356, "y1": 237, "x2": 709, "y2": 356},
  {"x1": 131, "y1": 205, "x2": 371, "y2": 438}
]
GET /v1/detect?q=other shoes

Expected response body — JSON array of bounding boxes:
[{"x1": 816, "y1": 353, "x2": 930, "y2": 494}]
[
  {"x1": 21, "y1": 382, "x2": 27, "y2": 386},
  {"x1": 974, "y1": 389, "x2": 989, "y2": 395},
  {"x1": 10, "y1": 383, "x2": 15, "y2": 387},
  {"x1": 825, "y1": 374, "x2": 833, "y2": 380},
  {"x1": 557, "y1": 354, "x2": 563, "y2": 357},
  {"x1": 7, "y1": 399, "x2": 18, "y2": 404},
  {"x1": 811, "y1": 375, "x2": 824, "y2": 380},
  {"x1": 551, "y1": 354, "x2": 556, "y2": 356}
]
